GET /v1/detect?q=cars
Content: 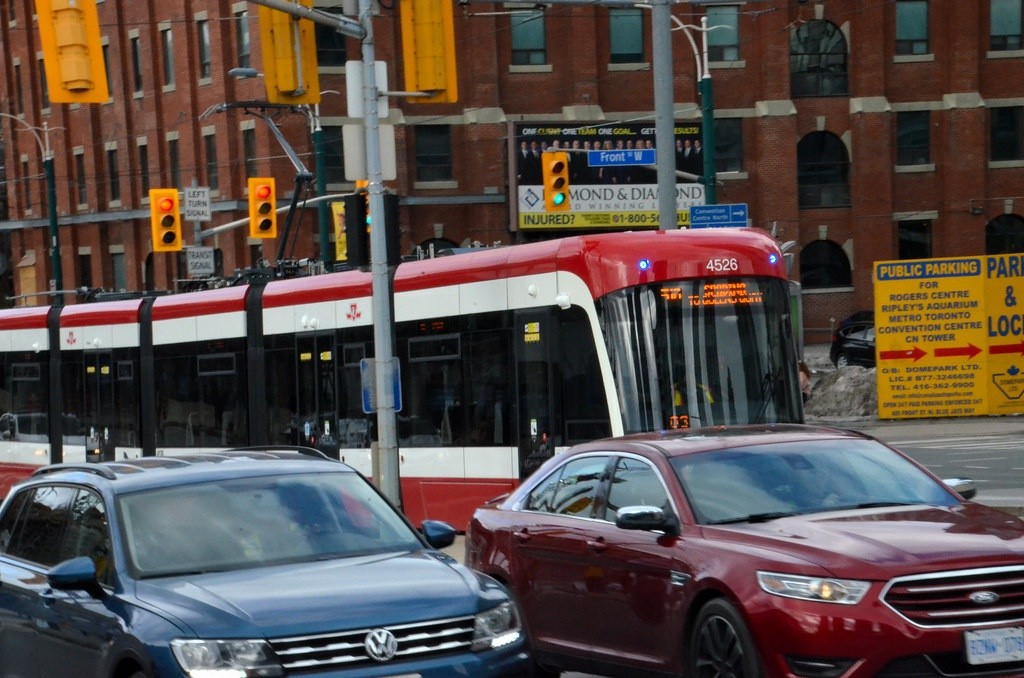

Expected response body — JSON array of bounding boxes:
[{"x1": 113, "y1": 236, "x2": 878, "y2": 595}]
[
  {"x1": 462, "y1": 422, "x2": 1023, "y2": 677},
  {"x1": 829, "y1": 308, "x2": 876, "y2": 370},
  {"x1": 1, "y1": 444, "x2": 535, "y2": 678}
]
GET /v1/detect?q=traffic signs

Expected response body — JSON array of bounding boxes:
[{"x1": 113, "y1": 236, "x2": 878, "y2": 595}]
[
  {"x1": 689, "y1": 203, "x2": 749, "y2": 229},
  {"x1": 871, "y1": 253, "x2": 1024, "y2": 419}
]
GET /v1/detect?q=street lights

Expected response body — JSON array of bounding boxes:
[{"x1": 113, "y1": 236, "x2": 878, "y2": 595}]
[
  {"x1": 228, "y1": 68, "x2": 342, "y2": 273},
  {"x1": 634, "y1": 4, "x2": 735, "y2": 206}
]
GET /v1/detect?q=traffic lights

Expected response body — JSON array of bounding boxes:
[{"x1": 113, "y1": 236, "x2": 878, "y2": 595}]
[
  {"x1": 541, "y1": 150, "x2": 570, "y2": 213},
  {"x1": 149, "y1": 188, "x2": 182, "y2": 253},
  {"x1": 247, "y1": 177, "x2": 277, "y2": 239}
]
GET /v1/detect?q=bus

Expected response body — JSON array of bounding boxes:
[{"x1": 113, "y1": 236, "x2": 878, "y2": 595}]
[{"x1": 0, "y1": 97, "x2": 807, "y2": 534}]
